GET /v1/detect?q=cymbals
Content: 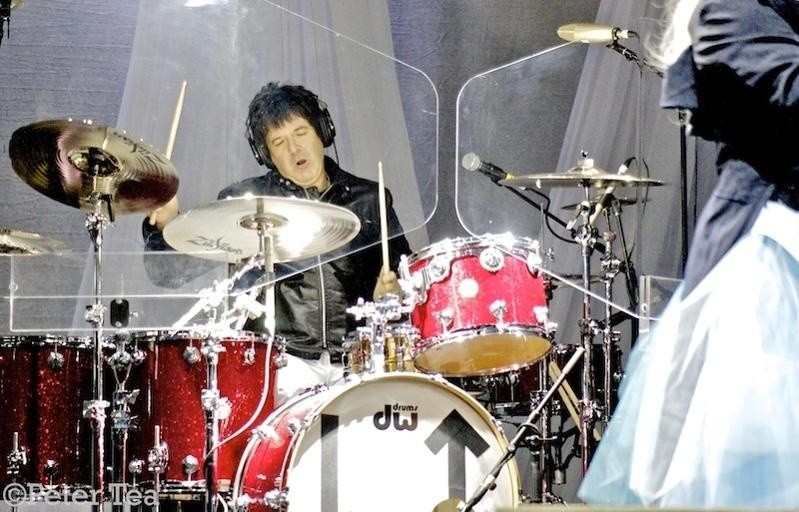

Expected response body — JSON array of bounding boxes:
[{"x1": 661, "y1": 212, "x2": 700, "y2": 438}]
[
  {"x1": 163, "y1": 195, "x2": 360, "y2": 266},
  {"x1": 8, "y1": 118, "x2": 179, "y2": 213},
  {"x1": 0, "y1": 227, "x2": 66, "y2": 256},
  {"x1": 504, "y1": 170, "x2": 664, "y2": 187}
]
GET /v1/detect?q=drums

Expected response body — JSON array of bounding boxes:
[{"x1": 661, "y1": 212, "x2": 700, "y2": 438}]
[
  {"x1": 401, "y1": 235, "x2": 553, "y2": 377},
  {"x1": 0, "y1": 335, "x2": 148, "y2": 499},
  {"x1": 144, "y1": 328, "x2": 281, "y2": 499},
  {"x1": 233, "y1": 371, "x2": 520, "y2": 509}
]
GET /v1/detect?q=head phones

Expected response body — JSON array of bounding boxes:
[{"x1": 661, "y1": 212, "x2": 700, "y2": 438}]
[{"x1": 244, "y1": 107, "x2": 336, "y2": 169}]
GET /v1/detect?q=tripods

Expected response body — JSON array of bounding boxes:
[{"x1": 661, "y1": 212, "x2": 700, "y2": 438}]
[{"x1": 458, "y1": 231, "x2": 616, "y2": 512}]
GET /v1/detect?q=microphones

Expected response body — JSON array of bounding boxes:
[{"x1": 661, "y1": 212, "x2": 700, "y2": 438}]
[
  {"x1": 556, "y1": 21, "x2": 633, "y2": 44},
  {"x1": 587, "y1": 158, "x2": 632, "y2": 228},
  {"x1": 462, "y1": 152, "x2": 517, "y2": 183}
]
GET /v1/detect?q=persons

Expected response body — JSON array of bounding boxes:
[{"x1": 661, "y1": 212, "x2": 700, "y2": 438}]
[
  {"x1": 574, "y1": 2, "x2": 799, "y2": 512},
  {"x1": 136, "y1": 82, "x2": 418, "y2": 418}
]
